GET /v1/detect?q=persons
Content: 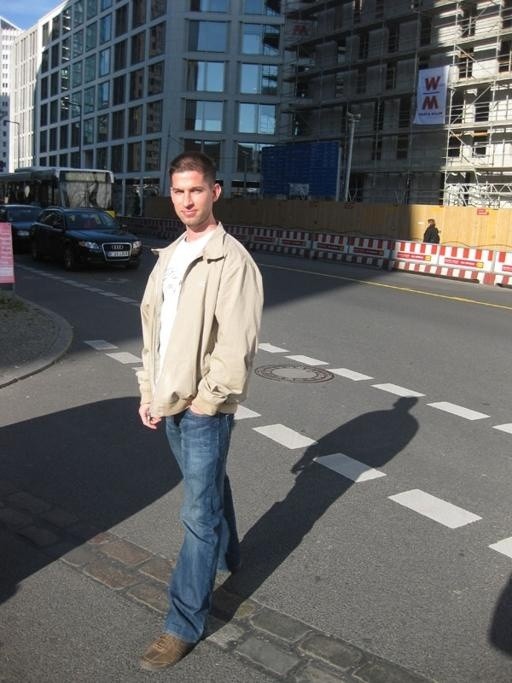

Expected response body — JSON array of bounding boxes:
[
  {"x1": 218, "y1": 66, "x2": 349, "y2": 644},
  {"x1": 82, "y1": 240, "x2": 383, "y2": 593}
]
[
  {"x1": 423, "y1": 219, "x2": 440, "y2": 244},
  {"x1": 138, "y1": 150, "x2": 264, "y2": 670}
]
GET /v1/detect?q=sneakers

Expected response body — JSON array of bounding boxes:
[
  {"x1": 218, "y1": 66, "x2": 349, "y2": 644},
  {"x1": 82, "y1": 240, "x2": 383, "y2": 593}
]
[
  {"x1": 214, "y1": 560, "x2": 245, "y2": 591},
  {"x1": 139, "y1": 633, "x2": 195, "y2": 672}
]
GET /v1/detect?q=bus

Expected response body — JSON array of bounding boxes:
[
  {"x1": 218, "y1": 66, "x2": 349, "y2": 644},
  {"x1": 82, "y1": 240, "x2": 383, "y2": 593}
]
[{"x1": 0, "y1": 166, "x2": 116, "y2": 227}]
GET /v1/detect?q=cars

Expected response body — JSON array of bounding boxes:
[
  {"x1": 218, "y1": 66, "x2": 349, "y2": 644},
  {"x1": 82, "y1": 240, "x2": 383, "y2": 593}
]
[
  {"x1": 29, "y1": 205, "x2": 143, "y2": 270},
  {"x1": 0, "y1": 205, "x2": 43, "y2": 253}
]
